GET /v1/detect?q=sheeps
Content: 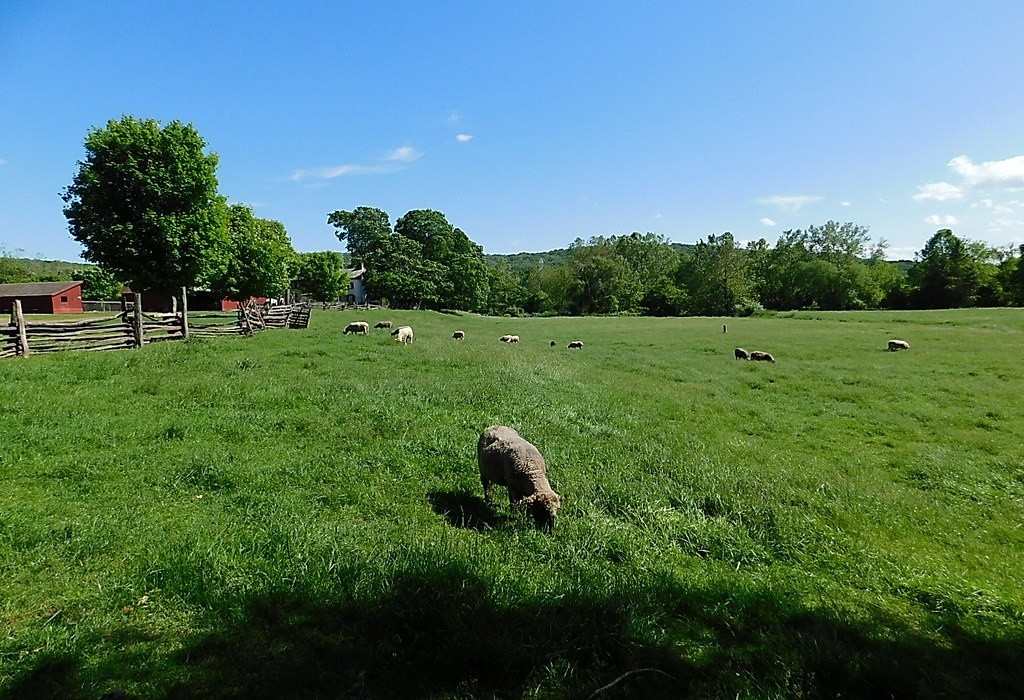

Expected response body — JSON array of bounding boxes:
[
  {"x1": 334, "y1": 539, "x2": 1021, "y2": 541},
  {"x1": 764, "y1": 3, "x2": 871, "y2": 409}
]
[
  {"x1": 499, "y1": 334, "x2": 520, "y2": 344},
  {"x1": 750, "y1": 350, "x2": 775, "y2": 363},
  {"x1": 549, "y1": 340, "x2": 556, "y2": 347},
  {"x1": 734, "y1": 348, "x2": 750, "y2": 362},
  {"x1": 390, "y1": 325, "x2": 416, "y2": 347},
  {"x1": 886, "y1": 339, "x2": 911, "y2": 352},
  {"x1": 342, "y1": 321, "x2": 370, "y2": 336},
  {"x1": 566, "y1": 341, "x2": 584, "y2": 350},
  {"x1": 475, "y1": 424, "x2": 563, "y2": 535},
  {"x1": 722, "y1": 323, "x2": 728, "y2": 333},
  {"x1": 373, "y1": 320, "x2": 393, "y2": 329},
  {"x1": 452, "y1": 330, "x2": 465, "y2": 340}
]
